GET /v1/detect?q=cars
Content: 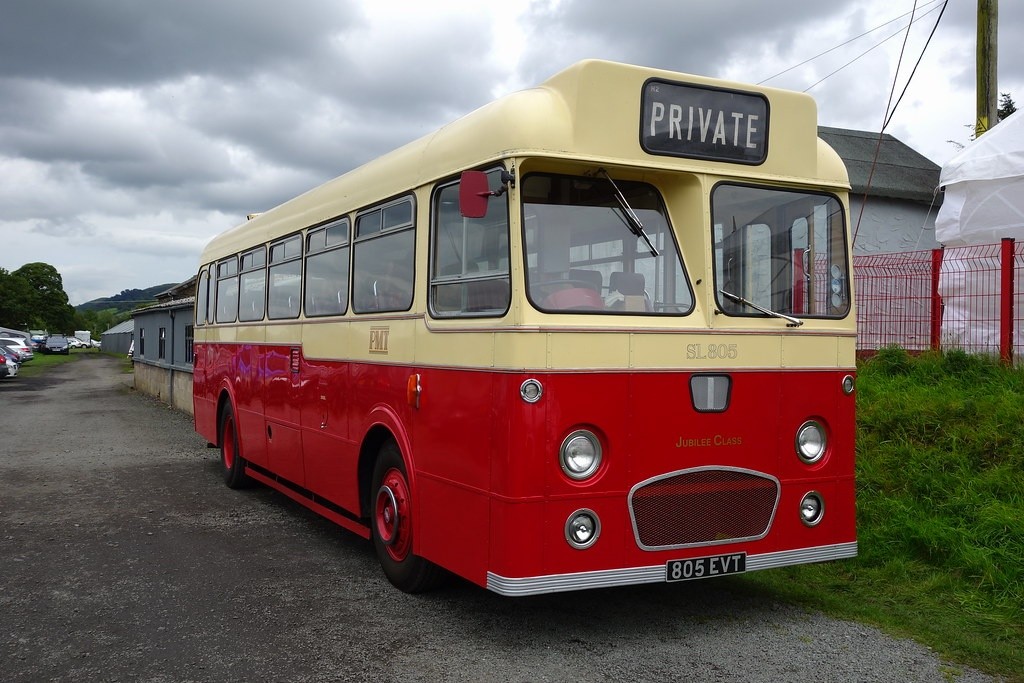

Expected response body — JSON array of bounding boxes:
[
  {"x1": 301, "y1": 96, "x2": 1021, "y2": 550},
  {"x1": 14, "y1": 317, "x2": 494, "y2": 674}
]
[
  {"x1": 0, "y1": 326, "x2": 47, "y2": 377},
  {"x1": 91, "y1": 340, "x2": 101, "y2": 348},
  {"x1": 67, "y1": 338, "x2": 82, "y2": 349},
  {"x1": 45, "y1": 333, "x2": 69, "y2": 355},
  {"x1": 66, "y1": 336, "x2": 92, "y2": 348}
]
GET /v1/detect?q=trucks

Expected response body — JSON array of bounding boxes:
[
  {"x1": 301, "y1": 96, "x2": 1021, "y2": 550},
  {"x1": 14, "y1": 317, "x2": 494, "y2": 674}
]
[{"x1": 74, "y1": 331, "x2": 90, "y2": 343}]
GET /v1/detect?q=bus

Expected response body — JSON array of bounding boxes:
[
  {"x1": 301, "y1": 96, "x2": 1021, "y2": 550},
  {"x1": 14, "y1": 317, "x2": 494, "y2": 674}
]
[{"x1": 192, "y1": 58, "x2": 858, "y2": 598}]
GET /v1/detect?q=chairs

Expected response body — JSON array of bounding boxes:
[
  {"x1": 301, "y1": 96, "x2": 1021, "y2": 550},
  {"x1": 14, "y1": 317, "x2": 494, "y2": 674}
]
[{"x1": 218, "y1": 261, "x2": 655, "y2": 321}]
